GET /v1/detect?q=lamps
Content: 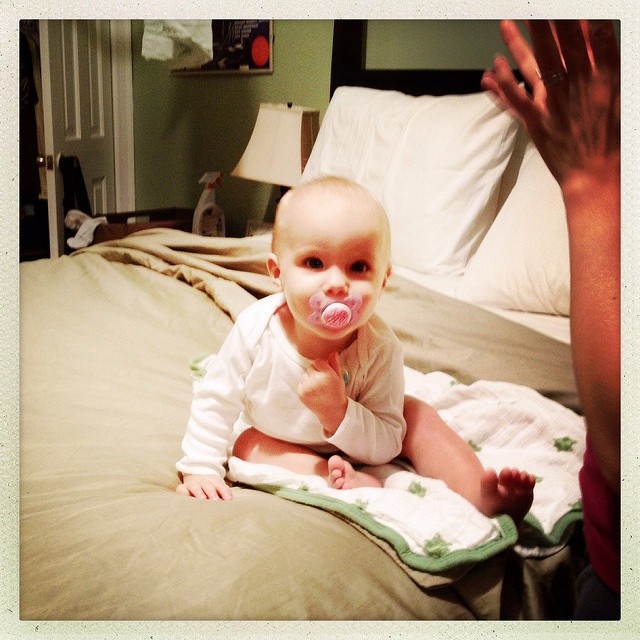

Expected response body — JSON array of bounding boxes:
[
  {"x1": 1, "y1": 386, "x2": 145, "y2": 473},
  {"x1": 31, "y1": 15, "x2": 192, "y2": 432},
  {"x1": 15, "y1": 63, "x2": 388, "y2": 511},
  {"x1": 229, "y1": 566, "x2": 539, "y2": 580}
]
[{"x1": 230, "y1": 103, "x2": 320, "y2": 197}]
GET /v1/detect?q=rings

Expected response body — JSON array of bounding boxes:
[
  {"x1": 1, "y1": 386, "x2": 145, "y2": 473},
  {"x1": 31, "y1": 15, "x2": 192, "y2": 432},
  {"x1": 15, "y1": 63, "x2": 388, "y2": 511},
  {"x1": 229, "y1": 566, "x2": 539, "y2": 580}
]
[{"x1": 541, "y1": 70, "x2": 569, "y2": 86}]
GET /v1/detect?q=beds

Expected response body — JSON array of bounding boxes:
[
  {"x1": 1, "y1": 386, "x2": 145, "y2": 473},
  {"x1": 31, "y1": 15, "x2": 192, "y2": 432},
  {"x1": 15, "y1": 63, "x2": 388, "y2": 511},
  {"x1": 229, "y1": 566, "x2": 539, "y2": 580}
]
[{"x1": 20, "y1": 227, "x2": 586, "y2": 620}]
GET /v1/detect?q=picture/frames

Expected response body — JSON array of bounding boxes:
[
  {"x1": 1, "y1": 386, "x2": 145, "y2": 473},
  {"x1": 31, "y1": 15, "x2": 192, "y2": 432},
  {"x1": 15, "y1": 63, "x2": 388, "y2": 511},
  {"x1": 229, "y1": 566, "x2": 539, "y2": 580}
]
[{"x1": 171, "y1": 19, "x2": 274, "y2": 77}]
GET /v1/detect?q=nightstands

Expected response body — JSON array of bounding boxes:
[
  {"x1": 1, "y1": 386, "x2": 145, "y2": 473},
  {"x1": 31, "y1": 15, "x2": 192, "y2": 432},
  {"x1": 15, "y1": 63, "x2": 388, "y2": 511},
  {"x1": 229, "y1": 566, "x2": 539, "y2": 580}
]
[{"x1": 86, "y1": 207, "x2": 195, "y2": 245}]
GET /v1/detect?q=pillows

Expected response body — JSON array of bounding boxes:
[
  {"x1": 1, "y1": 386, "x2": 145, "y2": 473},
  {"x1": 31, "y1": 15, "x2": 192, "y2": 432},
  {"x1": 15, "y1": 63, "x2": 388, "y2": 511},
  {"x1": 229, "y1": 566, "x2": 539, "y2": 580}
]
[
  {"x1": 293, "y1": 84, "x2": 521, "y2": 297},
  {"x1": 446, "y1": 123, "x2": 576, "y2": 316}
]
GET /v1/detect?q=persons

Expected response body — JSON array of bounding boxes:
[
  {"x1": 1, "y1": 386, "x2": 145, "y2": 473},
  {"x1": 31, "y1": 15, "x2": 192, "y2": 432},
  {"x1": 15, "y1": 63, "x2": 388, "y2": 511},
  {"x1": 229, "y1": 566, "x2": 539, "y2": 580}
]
[
  {"x1": 176, "y1": 176, "x2": 535, "y2": 525},
  {"x1": 482, "y1": 18, "x2": 620, "y2": 593}
]
